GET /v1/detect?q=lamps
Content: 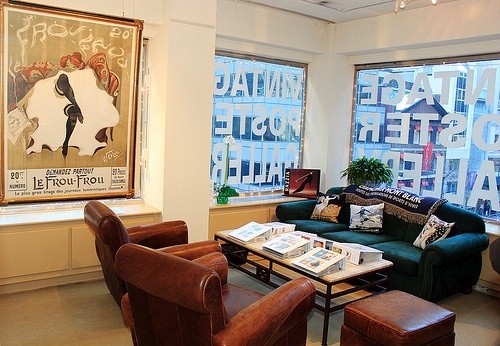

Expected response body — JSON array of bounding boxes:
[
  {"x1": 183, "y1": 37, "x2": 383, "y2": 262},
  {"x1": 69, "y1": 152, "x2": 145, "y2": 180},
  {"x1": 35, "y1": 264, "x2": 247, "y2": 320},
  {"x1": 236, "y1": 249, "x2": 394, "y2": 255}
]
[{"x1": 400, "y1": 0, "x2": 408, "y2": 9}]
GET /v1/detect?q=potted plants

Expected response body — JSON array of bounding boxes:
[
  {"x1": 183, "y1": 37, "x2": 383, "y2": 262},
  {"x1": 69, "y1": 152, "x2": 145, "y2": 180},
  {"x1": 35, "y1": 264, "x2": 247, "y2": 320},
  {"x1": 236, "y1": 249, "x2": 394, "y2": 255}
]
[{"x1": 340, "y1": 156, "x2": 395, "y2": 189}]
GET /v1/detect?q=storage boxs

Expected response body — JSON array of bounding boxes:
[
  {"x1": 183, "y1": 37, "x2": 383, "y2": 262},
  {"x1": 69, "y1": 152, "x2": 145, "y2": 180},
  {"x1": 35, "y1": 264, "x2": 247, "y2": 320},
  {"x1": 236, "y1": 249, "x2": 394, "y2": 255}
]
[{"x1": 282, "y1": 168, "x2": 321, "y2": 199}]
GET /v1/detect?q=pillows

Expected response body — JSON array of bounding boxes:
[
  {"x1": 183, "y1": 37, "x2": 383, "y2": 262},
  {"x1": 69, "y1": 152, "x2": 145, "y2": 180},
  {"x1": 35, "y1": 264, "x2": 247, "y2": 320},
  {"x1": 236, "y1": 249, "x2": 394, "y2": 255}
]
[
  {"x1": 311, "y1": 191, "x2": 346, "y2": 224},
  {"x1": 412, "y1": 214, "x2": 455, "y2": 249},
  {"x1": 348, "y1": 203, "x2": 384, "y2": 233}
]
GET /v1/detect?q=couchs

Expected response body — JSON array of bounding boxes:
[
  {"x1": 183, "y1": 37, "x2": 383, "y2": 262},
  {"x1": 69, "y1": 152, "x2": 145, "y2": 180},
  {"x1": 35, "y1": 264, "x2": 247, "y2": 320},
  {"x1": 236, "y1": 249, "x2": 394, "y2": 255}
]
[
  {"x1": 84, "y1": 201, "x2": 316, "y2": 346},
  {"x1": 276, "y1": 186, "x2": 489, "y2": 301}
]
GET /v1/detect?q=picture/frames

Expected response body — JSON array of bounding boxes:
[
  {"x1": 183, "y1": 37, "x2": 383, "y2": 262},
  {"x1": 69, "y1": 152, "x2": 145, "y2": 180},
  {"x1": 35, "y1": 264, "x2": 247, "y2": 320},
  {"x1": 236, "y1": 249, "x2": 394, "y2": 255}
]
[{"x1": 0, "y1": 0, "x2": 144, "y2": 206}]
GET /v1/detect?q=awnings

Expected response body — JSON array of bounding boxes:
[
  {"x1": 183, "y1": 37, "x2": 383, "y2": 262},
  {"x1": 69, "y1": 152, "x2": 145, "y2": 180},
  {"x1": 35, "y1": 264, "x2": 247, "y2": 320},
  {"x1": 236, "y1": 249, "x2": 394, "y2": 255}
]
[{"x1": 414, "y1": 124, "x2": 444, "y2": 132}]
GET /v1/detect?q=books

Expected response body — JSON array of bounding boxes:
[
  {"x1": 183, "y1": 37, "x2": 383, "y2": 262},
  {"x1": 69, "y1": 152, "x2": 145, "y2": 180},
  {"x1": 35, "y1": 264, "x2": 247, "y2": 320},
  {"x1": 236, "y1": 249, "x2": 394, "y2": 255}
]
[
  {"x1": 290, "y1": 230, "x2": 385, "y2": 266},
  {"x1": 290, "y1": 246, "x2": 346, "y2": 278},
  {"x1": 262, "y1": 233, "x2": 311, "y2": 260},
  {"x1": 228, "y1": 221, "x2": 272, "y2": 245},
  {"x1": 262, "y1": 222, "x2": 296, "y2": 234}
]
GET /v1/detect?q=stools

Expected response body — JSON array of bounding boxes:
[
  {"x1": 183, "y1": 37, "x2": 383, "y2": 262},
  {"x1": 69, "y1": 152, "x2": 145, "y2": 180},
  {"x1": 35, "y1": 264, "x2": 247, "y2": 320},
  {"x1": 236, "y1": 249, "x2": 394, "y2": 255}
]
[{"x1": 340, "y1": 290, "x2": 456, "y2": 346}]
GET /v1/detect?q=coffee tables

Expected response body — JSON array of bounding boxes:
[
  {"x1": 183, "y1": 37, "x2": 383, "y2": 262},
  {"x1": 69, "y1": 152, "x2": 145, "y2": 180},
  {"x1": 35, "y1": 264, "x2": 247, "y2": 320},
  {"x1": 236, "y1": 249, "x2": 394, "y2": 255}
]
[{"x1": 215, "y1": 229, "x2": 393, "y2": 346}]
